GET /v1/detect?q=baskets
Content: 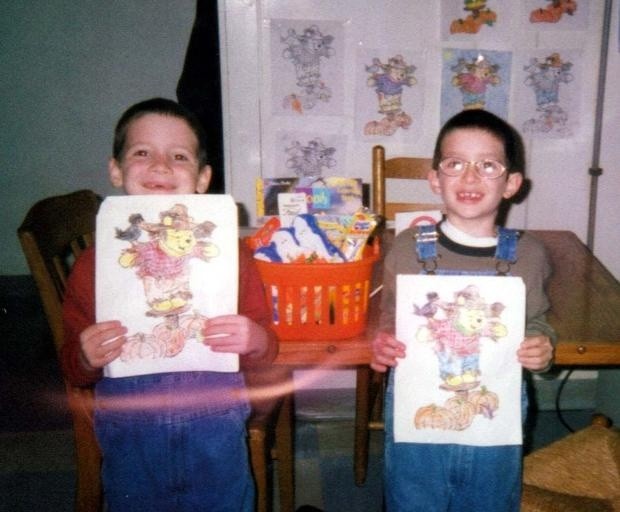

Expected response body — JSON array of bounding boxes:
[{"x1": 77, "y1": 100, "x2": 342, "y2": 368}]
[{"x1": 245, "y1": 214, "x2": 382, "y2": 345}]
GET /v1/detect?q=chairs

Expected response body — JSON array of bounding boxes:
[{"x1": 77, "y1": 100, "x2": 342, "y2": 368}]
[
  {"x1": 351, "y1": 146, "x2": 446, "y2": 482},
  {"x1": 18, "y1": 189, "x2": 298, "y2": 512}
]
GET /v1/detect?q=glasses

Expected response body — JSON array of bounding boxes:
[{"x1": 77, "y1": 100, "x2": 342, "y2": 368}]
[{"x1": 437, "y1": 155, "x2": 510, "y2": 179}]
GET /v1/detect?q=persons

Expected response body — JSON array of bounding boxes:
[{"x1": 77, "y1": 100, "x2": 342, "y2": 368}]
[
  {"x1": 368, "y1": 109, "x2": 557, "y2": 512},
  {"x1": 63, "y1": 96, "x2": 277, "y2": 511}
]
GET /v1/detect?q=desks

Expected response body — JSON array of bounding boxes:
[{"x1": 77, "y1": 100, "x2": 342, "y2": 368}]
[{"x1": 263, "y1": 229, "x2": 619, "y2": 374}]
